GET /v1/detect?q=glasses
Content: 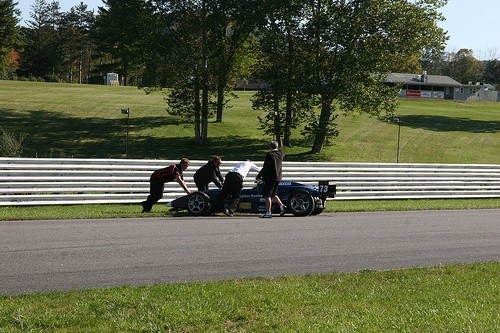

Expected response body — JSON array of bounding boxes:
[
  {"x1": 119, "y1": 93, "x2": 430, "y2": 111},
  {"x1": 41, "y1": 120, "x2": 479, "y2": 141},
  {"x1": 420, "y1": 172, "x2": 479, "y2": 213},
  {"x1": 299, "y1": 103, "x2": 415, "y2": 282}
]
[{"x1": 216, "y1": 162, "x2": 222, "y2": 164}]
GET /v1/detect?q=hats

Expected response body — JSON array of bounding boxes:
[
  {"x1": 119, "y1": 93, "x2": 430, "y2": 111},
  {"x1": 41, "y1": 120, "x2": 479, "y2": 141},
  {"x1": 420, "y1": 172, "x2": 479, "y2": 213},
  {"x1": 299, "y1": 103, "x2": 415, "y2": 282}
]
[{"x1": 270, "y1": 141, "x2": 279, "y2": 152}]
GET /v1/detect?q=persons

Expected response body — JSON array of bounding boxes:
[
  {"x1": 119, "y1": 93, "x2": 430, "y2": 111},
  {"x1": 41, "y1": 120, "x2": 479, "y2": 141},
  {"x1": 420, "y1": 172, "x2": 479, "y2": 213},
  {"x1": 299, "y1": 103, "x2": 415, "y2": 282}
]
[
  {"x1": 256, "y1": 141, "x2": 287, "y2": 218},
  {"x1": 140, "y1": 158, "x2": 192, "y2": 213},
  {"x1": 193, "y1": 157, "x2": 225, "y2": 192},
  {"x1": 223, "y1": 159, "x2": 261, "y2": 216}
]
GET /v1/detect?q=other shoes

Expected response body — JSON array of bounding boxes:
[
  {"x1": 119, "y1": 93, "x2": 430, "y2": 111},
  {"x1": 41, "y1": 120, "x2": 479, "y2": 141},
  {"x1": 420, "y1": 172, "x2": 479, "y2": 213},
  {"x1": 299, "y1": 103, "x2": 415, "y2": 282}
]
[
  {"x1": 259, "y1": 212, "x2": 272, "y2": 218},
  {"x1": 279, "y1": 205, "x2": 287, "y2": 216},
  {"x1": 223, "y1": 209, "x2": 233, "y2": 217}
]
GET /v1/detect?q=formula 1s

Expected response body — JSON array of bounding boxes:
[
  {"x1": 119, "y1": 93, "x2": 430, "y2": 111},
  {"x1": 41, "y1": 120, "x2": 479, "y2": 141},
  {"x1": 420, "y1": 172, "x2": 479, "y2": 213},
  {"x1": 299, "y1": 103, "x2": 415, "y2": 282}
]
[{"x1": 164, "y1": 176, "x2": 337, "y2": 217}]
